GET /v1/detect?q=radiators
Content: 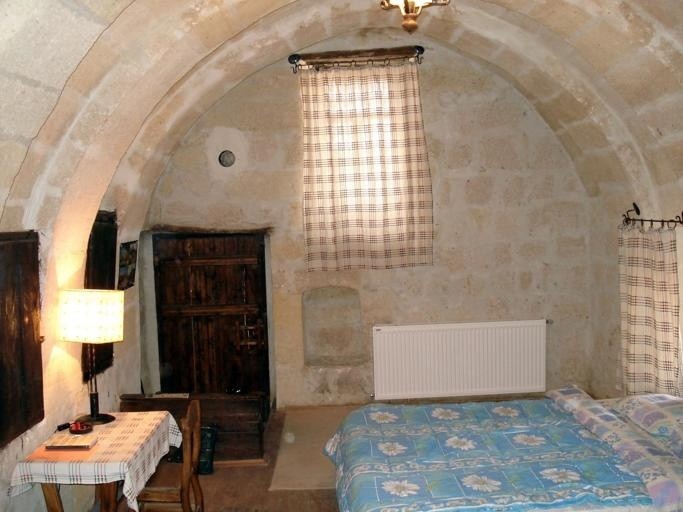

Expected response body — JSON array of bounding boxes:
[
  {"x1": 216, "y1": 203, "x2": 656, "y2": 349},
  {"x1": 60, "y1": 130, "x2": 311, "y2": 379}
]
[{"x1": 370, "y1": 318, "x2": 550, "y2": 399}]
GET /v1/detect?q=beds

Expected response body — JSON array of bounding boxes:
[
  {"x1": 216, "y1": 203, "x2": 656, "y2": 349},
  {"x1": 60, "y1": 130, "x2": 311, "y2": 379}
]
[{"x1": 336, "y1": 395, "x2": 683, "y2": 512}]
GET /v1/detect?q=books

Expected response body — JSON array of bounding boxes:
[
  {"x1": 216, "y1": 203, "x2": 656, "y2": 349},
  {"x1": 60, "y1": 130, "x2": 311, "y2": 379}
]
[{"x1": 44, "y1": 433, "x2": 98, "y2": 450}]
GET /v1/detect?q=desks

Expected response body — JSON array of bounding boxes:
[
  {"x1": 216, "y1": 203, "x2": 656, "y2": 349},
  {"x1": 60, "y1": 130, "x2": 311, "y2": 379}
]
[{"x1": 18, "y1": 410, "x2": 171, "y2": 511}]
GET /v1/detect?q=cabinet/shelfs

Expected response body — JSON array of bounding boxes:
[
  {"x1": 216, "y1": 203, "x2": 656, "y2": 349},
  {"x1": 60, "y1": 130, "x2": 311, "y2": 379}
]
[
  {"x1": 1, "y1": 231, "x2": 46, "y2": 449},
  {"x1": 154, "y1": 229, "x2": 276, "y2": 421}
]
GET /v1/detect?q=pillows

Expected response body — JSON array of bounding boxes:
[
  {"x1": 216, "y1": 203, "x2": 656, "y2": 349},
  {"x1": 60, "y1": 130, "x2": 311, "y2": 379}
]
[{"x1": 615, "y1": 391, "x2": 683, "y2": 459}]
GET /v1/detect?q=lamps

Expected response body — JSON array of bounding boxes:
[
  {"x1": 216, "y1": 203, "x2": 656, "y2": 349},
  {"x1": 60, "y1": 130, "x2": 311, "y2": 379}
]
[{"x1": 58, "y1": 287, "x2": 126, "y2": 425}]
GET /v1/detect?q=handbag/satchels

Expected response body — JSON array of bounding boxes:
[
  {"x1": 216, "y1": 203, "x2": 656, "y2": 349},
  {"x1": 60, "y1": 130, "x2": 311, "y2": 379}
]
[{"x1": 168, "y1": 426, "x2": 217, "y2": 475}]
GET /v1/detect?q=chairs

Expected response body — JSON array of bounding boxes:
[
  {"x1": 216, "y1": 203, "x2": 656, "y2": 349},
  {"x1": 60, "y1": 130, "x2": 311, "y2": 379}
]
[{"x1": 131, "y1": 398, "x2": 204, "y2": 511}]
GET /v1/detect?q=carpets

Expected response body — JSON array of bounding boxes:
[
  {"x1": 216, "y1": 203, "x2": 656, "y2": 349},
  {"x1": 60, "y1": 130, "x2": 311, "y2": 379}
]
[{"x1": 269, "y1": 398, "x2": 358, "y2": 492}]
